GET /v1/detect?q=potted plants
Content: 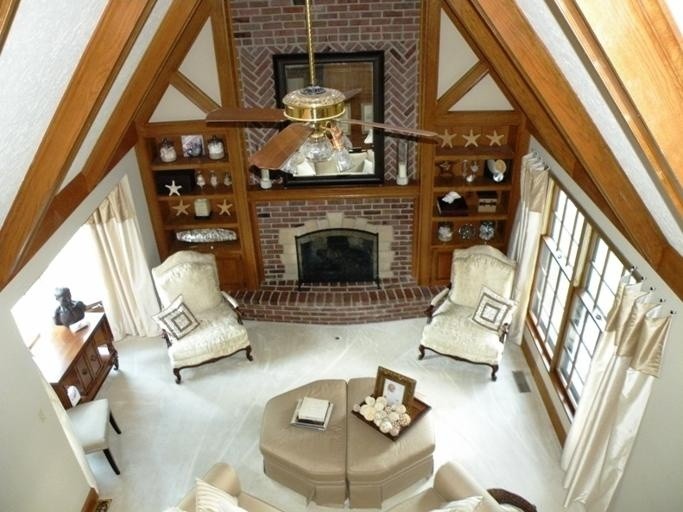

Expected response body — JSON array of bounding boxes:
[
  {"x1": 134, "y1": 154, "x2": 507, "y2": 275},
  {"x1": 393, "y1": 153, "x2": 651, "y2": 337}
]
[
  {"x1": 375, "y1": 364, "x2": 417, "y2": 414},
  {"x1": 271, "y1": 48, "x2": 383, "y2": 190}
]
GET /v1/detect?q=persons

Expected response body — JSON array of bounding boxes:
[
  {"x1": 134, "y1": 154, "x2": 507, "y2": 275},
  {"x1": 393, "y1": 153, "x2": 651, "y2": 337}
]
[{"x1": 50, "y1": 286, "x2": 87, "y2": 326}]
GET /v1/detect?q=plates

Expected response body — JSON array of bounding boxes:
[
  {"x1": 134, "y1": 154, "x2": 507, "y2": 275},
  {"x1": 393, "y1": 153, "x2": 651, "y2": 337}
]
[
  {"x1": 415, "y1": 109, "x2": 525, "y2": 292},
  {"x1": 52, "y1": 312, "x2": 120, "y2": 410},
  {"x1": 137, "y1": 122, "x2": 257, "y2": 293}
]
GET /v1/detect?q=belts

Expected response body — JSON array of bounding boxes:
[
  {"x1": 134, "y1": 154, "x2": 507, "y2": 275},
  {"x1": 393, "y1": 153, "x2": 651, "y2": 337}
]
[{"x1": 196, "y1": 170, "x2": 231, "y2": 194}]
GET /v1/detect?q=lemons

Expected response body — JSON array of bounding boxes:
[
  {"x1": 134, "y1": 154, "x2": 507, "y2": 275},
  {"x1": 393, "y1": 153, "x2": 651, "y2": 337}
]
[{"x1": 186, "y1": 144, "x2": 202, "y2": 158}]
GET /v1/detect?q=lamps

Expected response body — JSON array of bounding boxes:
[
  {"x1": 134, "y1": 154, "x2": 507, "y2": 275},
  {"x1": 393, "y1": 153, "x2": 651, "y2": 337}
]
[
  {"x1": 149, "y1": 249, "x2": 253, "y2": 384},
  {"x1": 417, "y1": 243, "x2": 517, "y2": 382}
]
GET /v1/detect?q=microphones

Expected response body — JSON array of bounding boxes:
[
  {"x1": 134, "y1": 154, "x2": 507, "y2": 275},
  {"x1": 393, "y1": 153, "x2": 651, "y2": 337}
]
[
  {"x1": 66, "y1": 399, "x2": 121, "y2": 477},
  {"x1": 259, "y1": 377, "x2": 436, "y2": 510}
]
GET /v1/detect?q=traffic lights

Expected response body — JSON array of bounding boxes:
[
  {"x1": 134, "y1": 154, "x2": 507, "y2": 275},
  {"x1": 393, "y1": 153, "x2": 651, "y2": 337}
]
[
  {"x1": 196, "y1": 478, "x2": 247, "y2": 512},
  {"x1": 151, "y1": 294, "x2": 205, "y2": 347},
  {"x1": 465, "y1": 285, "x2": 519, "y2": 337}
]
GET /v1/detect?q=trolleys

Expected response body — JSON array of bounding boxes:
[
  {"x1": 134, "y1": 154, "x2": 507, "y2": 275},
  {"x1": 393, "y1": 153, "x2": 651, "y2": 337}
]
[{"x1": 204, "y1": 3, "x2": 440, "y2": 170}]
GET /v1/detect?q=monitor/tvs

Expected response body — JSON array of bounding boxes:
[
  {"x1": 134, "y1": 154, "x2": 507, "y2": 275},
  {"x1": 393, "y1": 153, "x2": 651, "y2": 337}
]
[{"x1": 289, "y1": 396, "x2": 333, "y2": 432}]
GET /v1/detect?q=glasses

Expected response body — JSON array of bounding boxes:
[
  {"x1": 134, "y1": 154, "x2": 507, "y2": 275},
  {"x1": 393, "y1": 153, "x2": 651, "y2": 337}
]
[{"x1": 303, "y1": 133, "x2": 333, "y2": 165}]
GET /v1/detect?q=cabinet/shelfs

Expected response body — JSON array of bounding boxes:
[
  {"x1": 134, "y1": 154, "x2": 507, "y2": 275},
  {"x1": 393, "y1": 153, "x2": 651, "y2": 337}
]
[
  {"x1": 206, "y1": 135, "x2": 224, "y2": 160},
  {"x1": 158, "y1": 138, "x2": 177, "y2": 163}
]
